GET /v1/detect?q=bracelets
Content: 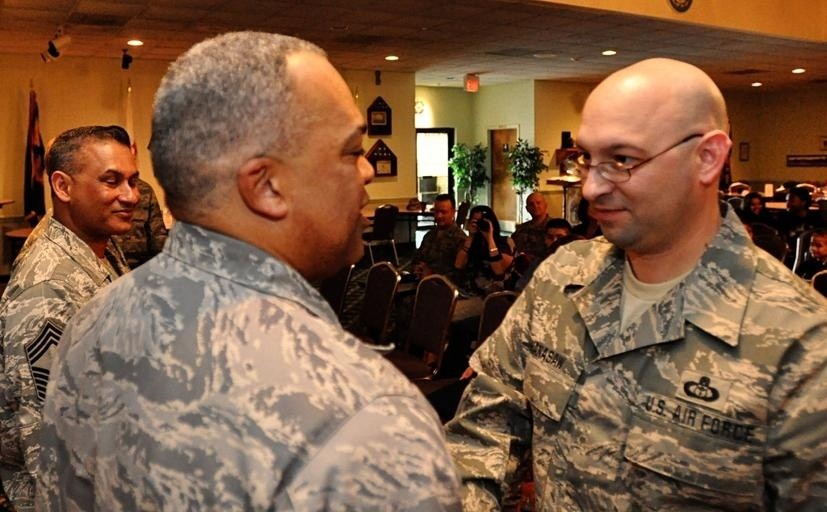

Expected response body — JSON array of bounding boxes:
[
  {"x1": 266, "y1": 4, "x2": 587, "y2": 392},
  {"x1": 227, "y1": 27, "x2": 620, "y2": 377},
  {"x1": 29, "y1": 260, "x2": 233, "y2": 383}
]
[
  {"x1": 490, "y1": 252, "x2": 502, "y2": 262},
  {"x1": 490, "y1": 248, "x2": 498, "y2": 252},
  {"x1": 462, "y1": 245, "x2": 470, "y2": 253}
]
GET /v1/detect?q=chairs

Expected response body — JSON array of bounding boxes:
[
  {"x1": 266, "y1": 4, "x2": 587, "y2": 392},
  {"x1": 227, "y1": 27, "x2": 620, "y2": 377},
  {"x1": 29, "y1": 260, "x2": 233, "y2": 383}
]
[
  {"x1": 338, "y1": 197, "x2": 527, "y2": 399},
  {"x1": 729, "y1": 180, "x2": 826, "y2": 275}
]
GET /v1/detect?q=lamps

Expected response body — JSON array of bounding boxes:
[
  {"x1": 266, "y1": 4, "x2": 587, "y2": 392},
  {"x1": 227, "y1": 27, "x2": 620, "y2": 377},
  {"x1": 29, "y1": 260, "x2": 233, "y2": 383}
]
[
  {"x1": 464, "y1": 74, "x2": 479, "y2": 93},
  {"x1": 40, "y1": 25, "x2": 72, "y2": 64}
]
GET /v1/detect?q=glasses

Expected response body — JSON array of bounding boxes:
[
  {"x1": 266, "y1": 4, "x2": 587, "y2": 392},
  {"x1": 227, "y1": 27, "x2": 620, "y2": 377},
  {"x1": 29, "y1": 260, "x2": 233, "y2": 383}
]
[{"x1": 563, "y1": 134, "x2": 703, "y2": 183}]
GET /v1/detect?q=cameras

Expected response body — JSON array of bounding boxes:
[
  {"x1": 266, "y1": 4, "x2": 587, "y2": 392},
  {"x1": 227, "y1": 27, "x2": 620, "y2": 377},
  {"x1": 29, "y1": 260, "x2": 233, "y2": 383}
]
[{"x1": 475, "y1": 212, "x2": 489, "y2": 232}]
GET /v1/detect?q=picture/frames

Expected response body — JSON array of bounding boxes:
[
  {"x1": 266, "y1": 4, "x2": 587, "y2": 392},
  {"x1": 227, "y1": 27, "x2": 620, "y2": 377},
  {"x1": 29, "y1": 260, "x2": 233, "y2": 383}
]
[{"x1": 739, "y1": 143, "x2": 749, "y2": 161}]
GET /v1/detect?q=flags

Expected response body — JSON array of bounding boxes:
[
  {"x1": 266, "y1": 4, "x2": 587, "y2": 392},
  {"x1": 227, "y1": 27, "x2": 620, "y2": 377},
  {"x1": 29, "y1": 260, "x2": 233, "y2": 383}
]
[{"x1": 24, "y1": 85, "x2": 45, "y2": 226}]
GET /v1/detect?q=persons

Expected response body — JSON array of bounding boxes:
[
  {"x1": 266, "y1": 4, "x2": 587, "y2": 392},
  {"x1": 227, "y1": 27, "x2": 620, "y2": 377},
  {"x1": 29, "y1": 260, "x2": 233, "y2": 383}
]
[
  {"x1": 573, "y1": 193, "x2": 603, "y2": 239},
  {"x1": 510, "y1": 193, "x2": 553, "y2": 250},
  {"x1": 453, "y1": 205, "x2": 514, "y2": 296},
  {"x1": 35, "y1": 32, "x2": 465, "y2": 512},
  {"x1": 442, "y1": 58, "x2": 827, "y2": 512},
  {"x1": 109, "y1": 125, "x2": 169, "y2": 272},
  {"x1": 384, "y1": 193, "x2": 467, "y2": 344},
  {"x1": 0, "y1": 126, "x2": 141, "y2": 512},
  {"x1": 728, "y1": 181, "x2": 827, "y2": 298},
  {"x1": 544, "y1": 218, "x2": 573, "y2": 247}
]
